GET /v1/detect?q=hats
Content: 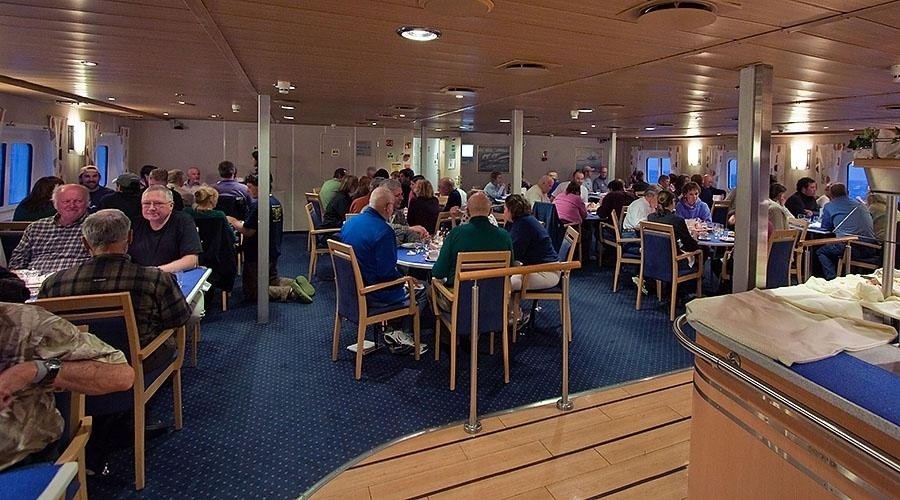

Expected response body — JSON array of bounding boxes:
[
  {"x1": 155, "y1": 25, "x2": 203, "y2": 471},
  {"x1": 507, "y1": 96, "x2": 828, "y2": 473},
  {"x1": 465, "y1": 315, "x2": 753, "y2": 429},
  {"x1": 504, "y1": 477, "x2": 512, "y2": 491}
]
[{"x1": 78, "y1": 164, "x2": 103, "y2": 175}]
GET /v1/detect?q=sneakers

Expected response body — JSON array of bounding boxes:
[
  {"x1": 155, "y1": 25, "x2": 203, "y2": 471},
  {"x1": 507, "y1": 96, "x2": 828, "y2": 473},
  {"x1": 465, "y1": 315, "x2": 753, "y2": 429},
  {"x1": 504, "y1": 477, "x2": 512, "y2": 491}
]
[
  {"x1": 291, "y1": 281, "x2": 313, "y2": 303},
  {"x1": 297, "y1": 275, "x2": 317, "y2": 296},
  {"x1": 391, "y1": 342, "x2": 431, "y2": 356},
  {"x1": 632, "y1": 274, "x2": 650, "y2": 295},
  {"x1": 383, "y1": 328, "x2": 415, "y2": 354},
  {"x1": 517, "y1": 313, "x2": 533, "y2": 336}
]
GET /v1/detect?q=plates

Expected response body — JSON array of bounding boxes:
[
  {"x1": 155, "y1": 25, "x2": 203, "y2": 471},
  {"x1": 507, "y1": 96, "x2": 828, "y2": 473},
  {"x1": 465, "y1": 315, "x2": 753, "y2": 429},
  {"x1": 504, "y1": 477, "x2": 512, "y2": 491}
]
[
  {"x1": 698, "y1": 237, "x2": 710, "y2": 240},
  {"x1": 401, "y1": 243, "x2": 421, "y2": 248},
  {"x1": 720, "y1": 237, "x2": 734, "y2": 242}
]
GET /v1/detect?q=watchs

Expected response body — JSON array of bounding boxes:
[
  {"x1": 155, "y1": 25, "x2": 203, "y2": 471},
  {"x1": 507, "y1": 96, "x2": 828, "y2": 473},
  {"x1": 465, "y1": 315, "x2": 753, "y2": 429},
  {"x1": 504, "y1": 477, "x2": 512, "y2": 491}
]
[{"x1": 39, "y1": 357, "x2": 62, "y2": 389}]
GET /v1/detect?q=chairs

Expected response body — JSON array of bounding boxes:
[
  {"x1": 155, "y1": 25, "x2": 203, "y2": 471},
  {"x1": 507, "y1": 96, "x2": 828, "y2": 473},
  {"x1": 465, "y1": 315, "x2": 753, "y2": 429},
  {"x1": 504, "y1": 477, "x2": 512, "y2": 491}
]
[
  {"x1": 345, "y1": 213, "x2": 359, "y2": 222},
  {"x1": 710, "y1": 200, "x2": 732, "y2": 257},
  {"x1": 611, "y1": 209, "x2": 659, "y2": 297},
  {"x1": 0, "y1": 221, "x2": 33, "y2": 269},
  {"x1": 327, "y1": 238, "x2": 420, "y2": 380},
  {"x1": 533, "y1": 201, "x2": 582, "y2": 263},
  {"x1": 433, "y1": 212, "x2": 463, "y2": 234},
  {"x1": 787, "y1": 218, "x2": 810, "y2": 284},
  {"x1": 598, "y1": 206, "x2": 629, "y2": 266},
  {"x1": 30, "y1": 324, "x2": 92, "y2": 500},
  {"x1": 836, "y1": 221, "x2": 900, "y2": 277},
  {"x1": 635, "y1": 220, "x2": 703, "y2": 321},
  {"x1": 513, "y1": 225, "x2": 579, "y2": 343},
  {"x1": 193, "y1": 216, "x2": 227, "y2": 311},
  {"x1": 721, "y1": 228, "x2": 801, "y2": 287},
  {"x1": 313, "y1": 186, "x2": 322, "y2": 194},
  {"x1": 304, "y1": 192, "x2": 325, "y2": 252},
  {"x1": 492, "y1": 212, "x2": 506, "y2": 228},
  {"x1": 431, "y1": 250, "x2": 510, "y2": 391},
  {"x1": 304, "y1": 202, "x2": 341, "y2": 282},
  {"x1": 213, "y1": 195, "x2": 247, "y2": 221},
  {"x1": 438, "y1": 195, "x2": 449, "y2": 209},
  {"x1": 471, "y1": 189, "x2": 484, "y2": 194},
  {"x1": 24, "y1": 291, "x2": 182, "y2": 490}
]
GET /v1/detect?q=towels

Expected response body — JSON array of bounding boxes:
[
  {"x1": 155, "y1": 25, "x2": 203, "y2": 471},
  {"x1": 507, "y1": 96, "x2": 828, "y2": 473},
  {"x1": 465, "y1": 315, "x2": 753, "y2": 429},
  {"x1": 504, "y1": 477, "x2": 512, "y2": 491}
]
[{"x1": 688, "y1": 283, "x2": 898, "y2": 366}]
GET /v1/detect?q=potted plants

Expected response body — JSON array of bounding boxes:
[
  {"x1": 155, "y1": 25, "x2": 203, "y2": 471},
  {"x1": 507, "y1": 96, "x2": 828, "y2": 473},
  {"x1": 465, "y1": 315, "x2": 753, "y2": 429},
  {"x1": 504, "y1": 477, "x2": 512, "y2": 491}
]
[{"x1": 846, "y1": 127, "x2": 880, "y2": 159}]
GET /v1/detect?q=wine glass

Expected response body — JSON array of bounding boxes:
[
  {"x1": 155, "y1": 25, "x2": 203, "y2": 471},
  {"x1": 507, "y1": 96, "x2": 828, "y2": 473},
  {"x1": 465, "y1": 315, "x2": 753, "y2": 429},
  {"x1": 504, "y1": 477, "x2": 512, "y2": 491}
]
[{"x1": 712, "y1": 222, "x2": 719, "y2": 242}]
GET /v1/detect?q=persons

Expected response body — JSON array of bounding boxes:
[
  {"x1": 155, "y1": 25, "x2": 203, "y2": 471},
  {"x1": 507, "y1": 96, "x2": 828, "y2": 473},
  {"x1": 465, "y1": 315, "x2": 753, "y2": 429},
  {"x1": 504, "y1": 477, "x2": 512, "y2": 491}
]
[
  {"x1": 369, "y1": 178, "x2": 385, "y2": 195},
  {"x1": 816, "y1": 184, "x2": 873, "y2": 281},
  {"x1": 9, "y1": 183, "x2": 93, "y2": 270},
  {"x1": 225, "y1": 172, "x2": 315, "y2": 304},
  {"x1": 817, "y1": 181, "x2": 836, "y2": 206},
  {"x1": 37, "y1": 209, "x2": 192, "y2": 456},
  {"x1": 691, "y1": 174, "x2": 703, "y2": 190},
  {"x1": 142, "y1": 168, "x2": 183, "y2": 210},
  {"x1": 673, "y1": 175, "x2": 691, "y2": 199},
  {"x1": 524, "y1": 175, "x2": 554, "y2": 213},
  {"x1": 140, "y1": 165, "x2": 159, "y2": 185},
  {"x1": 340, "y1": 186, "x2": 429, "y2": 357},
  {"x1": 210, "y1": 161, "x2": 252, "y2": 225},
  {"x1": 643, "y1": 175, "x2": 669, "y2": 193},
  {"x1": 769, "y1": 183, "x2": 796, "y2": 229},
  {"x1": 594, "y1": 180, "x2": 639, "y2": 264},
  {"x1": 582, "y1": 166, "x2": 594, "y2": 192},
  {"x1": 397, "y1": 168, "x2": 414, "y2": 209},
  {"x1": 722, "y1": 181, "x2": 737, "y2": 283},
  {"x1": 430, "y1": 192, "x2": 514, "y2": 346},
  {"x1": 667, "y1": 172, "x2": 678, "y2": 194},
  {"x1": 552, "y1": 180, "x2": 588, "y2": 264},
  {"x1": 592, "y1": 166, "x2": 610, "y2": 192},
  {"x1": 406, "y1": 180, "x2": 439, "y2": 235},
  {"x1": 128, "y1": 184, "x2": 203, "y2": 273},
  {"x1": 318, "y1": 169, "x2": 348, "y2": 210},
  {"x1": 483, "y1": 171, "x2": 506, "y2": 211},
  {"x1": 450, "y1": 191, "x2": 499, "y2": 227},
  {"x1": 675, "y1": 183, "x2": 710, "y2": 281},
  {"x1": 184, "y1": 166, "x2": 201, "y2": 190},
  {"x1": 783, "y1": 178, "x2": 821, "y2": 267},
  {"x1": 97, "y1": 173, "x2": 142, "y2": 210},
  {"x1": 549, "y1": 169, "x2": 592, "y2": 254},
  {"x1": 410, "y1": 175, "x2": 425, "y2": 189},
  {"x1": 621, "y1": 188, "x2": 662, "y2": 295},
  {"x1": 366, "y1": 166, "x2": 377, "y2": 178},
  {"x1": 866, "y1": 193, "x2": 900, "y2": 242},
  {"x1": 647, "y1": 191, "x2": 710, "y2": 299},
  {"x1": 438, "y1": 177, "x2": 467, "y2": 231},
  {"x1": 319, "y1": 175, "x2": 359, "y2": 249},
  {"x1": 629, "y1": 171, "x2": 650, "y2": 190},
  {"x1": 503, "y1": 194, "x2": 562, "y2": 332},
  {"x1": 183, "y1": 186, "x2": 238, "y2": 309},
  {"x1": 390, "y1": 172, "x2": 400, "y2": 183},
  {"x1": 359, "y1": 180, "x2": 429, "y2": 275},
  {"x1": 549, "y1": 172, "x2": 559, "y2": 197},
  {"x1": 79, "y1": 165, "x2": 115, "y2": 213},
  {"x1": 166, "y1": 169, "x2": 194, "y2": 204},
  {"x1": 698, "y1": 174, "x2": 726, "y2": 212},
  {"x1": 507, "y1": 168, "x2": 533, "y2": 191},
  {"x1": 1, "y1": 266, "x2": 134, "y2": 471},
  {"x1": 216, "y1": 165, "x2": 244, "y2": 183}
]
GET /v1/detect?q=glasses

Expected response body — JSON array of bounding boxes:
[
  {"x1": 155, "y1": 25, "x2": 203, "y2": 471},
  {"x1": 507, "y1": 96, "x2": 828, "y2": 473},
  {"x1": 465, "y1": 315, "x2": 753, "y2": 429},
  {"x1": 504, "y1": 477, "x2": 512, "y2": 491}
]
[
  {"x1": 143, "y1": 198, "x2": 173, "y2": 209},
  {"x1": 394, "y1": 192, "x2": 405, "y2": 197}
]
